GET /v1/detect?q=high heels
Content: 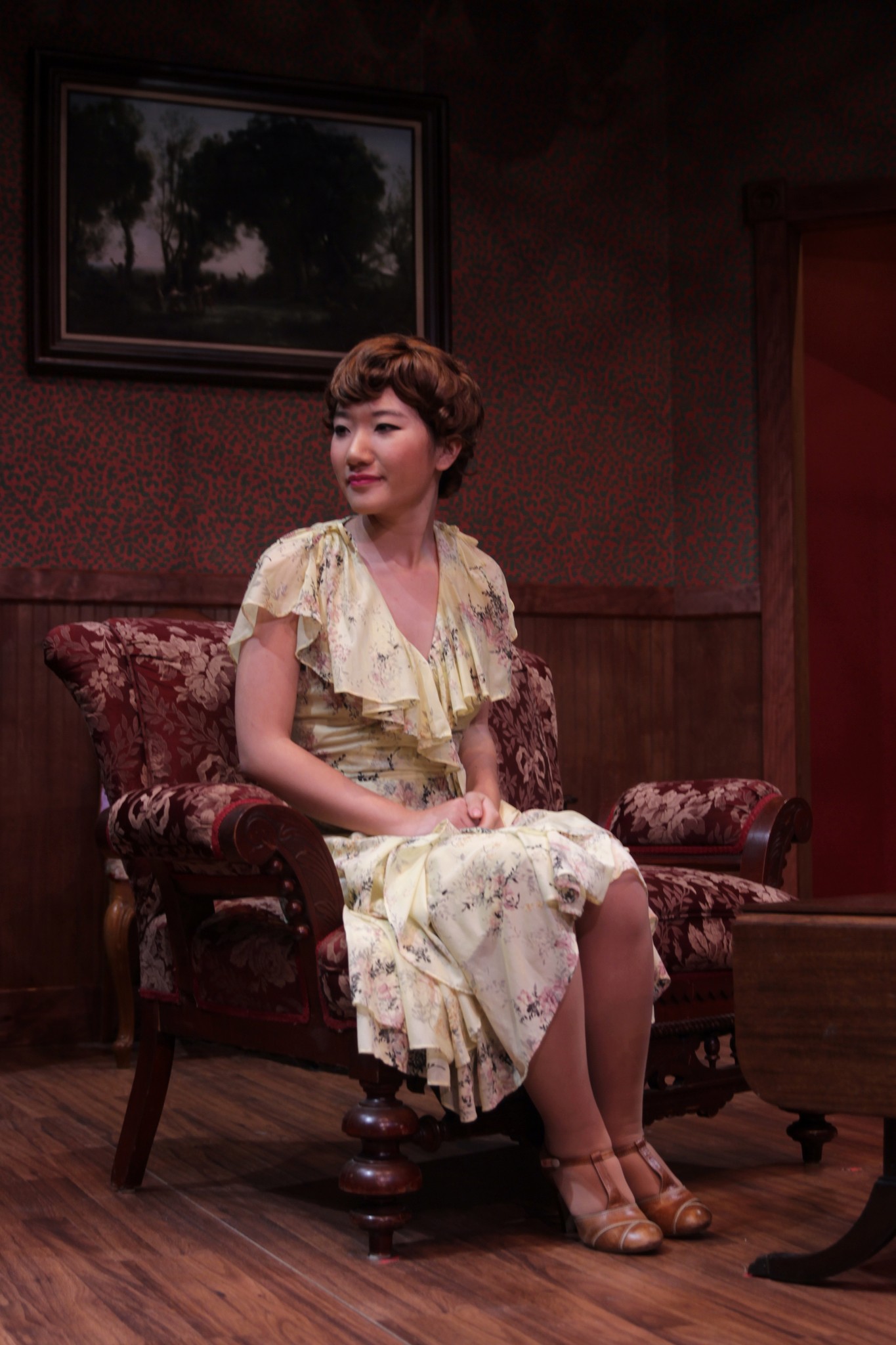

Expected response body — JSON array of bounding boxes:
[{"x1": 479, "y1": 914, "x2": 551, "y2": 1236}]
[{"x1": 541, "y1": 1133, "x2": 713, "y2": 1255}]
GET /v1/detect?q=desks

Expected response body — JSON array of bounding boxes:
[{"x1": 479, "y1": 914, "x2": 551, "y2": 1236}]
[{"x1": 727, "y1": 889, "x2": 896, "y2": 1282}]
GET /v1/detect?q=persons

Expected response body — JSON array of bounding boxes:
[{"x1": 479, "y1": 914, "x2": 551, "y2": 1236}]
[{"x1": 227, "y1": 336, "x2": 713, "y2": 1256}]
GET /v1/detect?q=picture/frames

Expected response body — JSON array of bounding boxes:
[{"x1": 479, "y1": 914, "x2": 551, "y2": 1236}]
[{"x1": 15, "y1": 37, "x2": 455, "y2": 394}]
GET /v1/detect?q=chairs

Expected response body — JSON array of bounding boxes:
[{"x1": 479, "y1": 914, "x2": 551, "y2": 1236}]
[{"x1": 43, "y1": 617, "x2": 837, "y2": 1261}]
